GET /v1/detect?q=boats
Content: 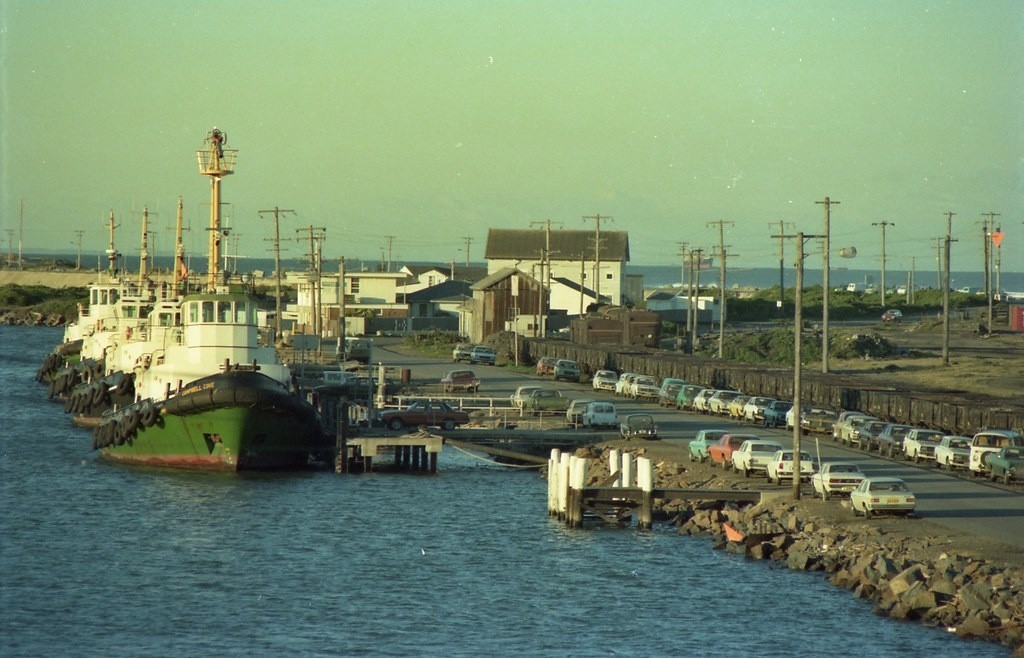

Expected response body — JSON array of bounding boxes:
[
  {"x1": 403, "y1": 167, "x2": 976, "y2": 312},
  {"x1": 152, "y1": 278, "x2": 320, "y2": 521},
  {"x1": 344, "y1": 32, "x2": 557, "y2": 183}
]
[{"x1": 36, "y1": 124, "x2": 336, "y2": 471}]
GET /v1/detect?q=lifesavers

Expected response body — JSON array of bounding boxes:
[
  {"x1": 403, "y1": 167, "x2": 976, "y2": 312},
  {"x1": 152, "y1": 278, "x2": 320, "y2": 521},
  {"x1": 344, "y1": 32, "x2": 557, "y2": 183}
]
[{"x1": 126, "y1": 326, "x2": 131, "y2": 340}]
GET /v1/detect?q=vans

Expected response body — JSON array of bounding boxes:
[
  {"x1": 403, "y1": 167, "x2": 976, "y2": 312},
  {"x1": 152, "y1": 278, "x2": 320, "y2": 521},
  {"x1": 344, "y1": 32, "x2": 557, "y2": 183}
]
[
  {"x1": 337, "y1": 337, "x2": 372, "y2": 361},
  {"x1": 322, "y1": 369, "x2": 359, "y2": 387}
]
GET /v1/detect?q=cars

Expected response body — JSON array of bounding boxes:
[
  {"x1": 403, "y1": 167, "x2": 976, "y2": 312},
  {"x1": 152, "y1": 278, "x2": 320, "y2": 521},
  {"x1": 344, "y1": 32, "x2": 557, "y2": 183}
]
[
  {"x1": 343, "y1": 378, "x2": 402, "y2": 401},
  {"x1": 846, "y1": 274, "x2": 1018, "y2": 302},
  {"x1": 380, "y1": 399, "x2": 469, "y2": 430},
  {"x1": 441, "y1": 340, "x2": 1024, "y2": 518},
  {"x1": 884, "y1": 310, "x2": 902, "y2": 322}
]
